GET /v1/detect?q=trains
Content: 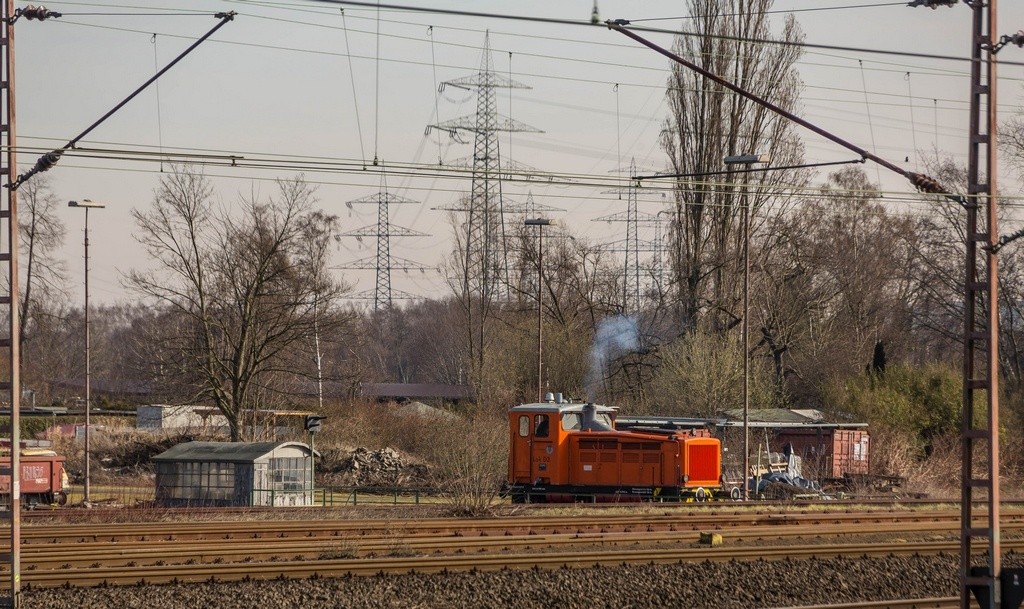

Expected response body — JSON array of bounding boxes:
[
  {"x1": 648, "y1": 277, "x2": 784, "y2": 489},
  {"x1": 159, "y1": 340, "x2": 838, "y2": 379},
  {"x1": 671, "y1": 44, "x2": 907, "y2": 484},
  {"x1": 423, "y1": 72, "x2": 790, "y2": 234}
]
[
  {"x1": 500, "y1": 391, "x2": 742, "y2": 502},
  {"x1": 0, "y1": 438, "x2": 70, "y2": 507}
]
